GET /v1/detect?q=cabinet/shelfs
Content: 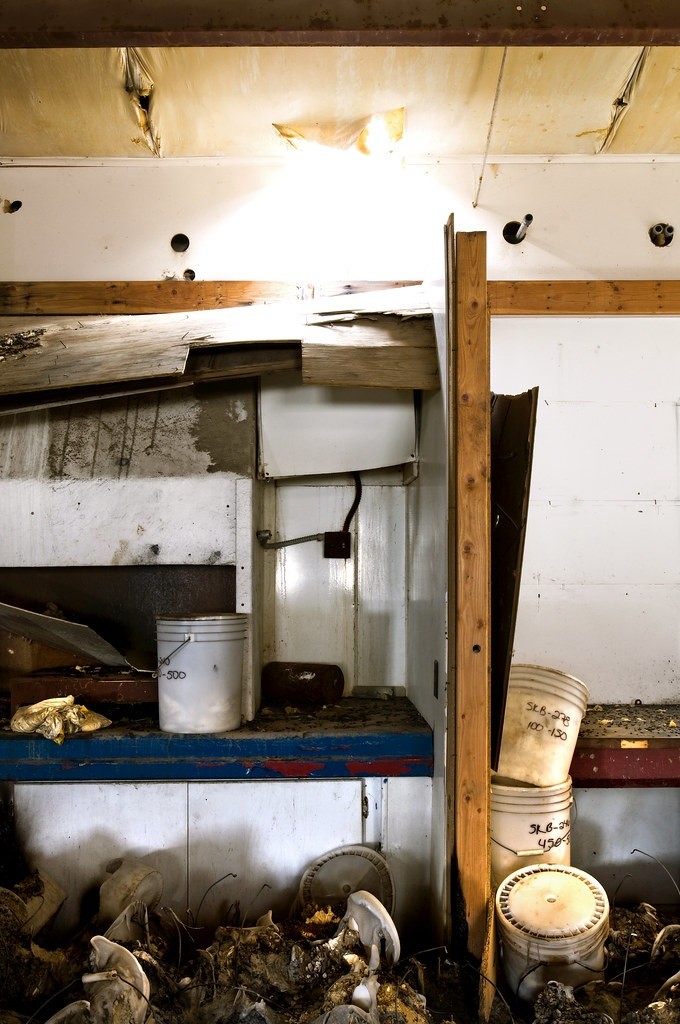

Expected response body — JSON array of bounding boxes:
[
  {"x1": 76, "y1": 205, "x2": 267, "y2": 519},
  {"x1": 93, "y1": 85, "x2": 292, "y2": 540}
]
[{"x1": 0, "y1": 213, "x2": 680, "y2": 1024}]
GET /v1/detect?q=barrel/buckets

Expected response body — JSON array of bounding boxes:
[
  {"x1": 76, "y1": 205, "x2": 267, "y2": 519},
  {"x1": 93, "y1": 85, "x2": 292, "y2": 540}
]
[
  {"x1": 488, "y1": 663, "x2": 588, "y2": 788},
  {"x1": 153, "y1": 613, "x2": 248, "y2": 733},
  {"x1": 487, "y1": 777, "x2": 573, "y2": 886},
  {"x1": 494, "y1": 863, "x2": 610, "y2": 998}
]
